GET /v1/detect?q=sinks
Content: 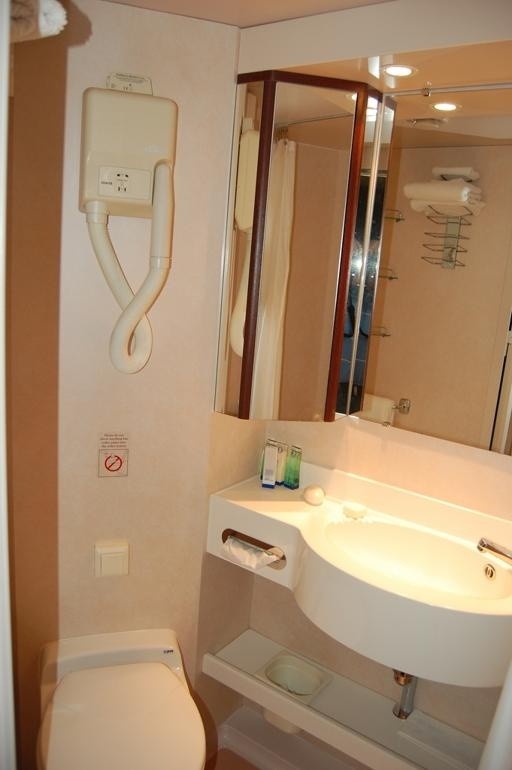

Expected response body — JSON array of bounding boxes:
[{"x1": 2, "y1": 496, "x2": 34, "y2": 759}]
[{"x1": 324, "y1": 521, "x2": 512, "y2": 601}]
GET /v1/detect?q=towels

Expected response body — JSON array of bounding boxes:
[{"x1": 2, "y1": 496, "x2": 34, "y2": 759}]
[
  {"x1": 360, "y1": 392, "x2": 399, "y2": 425},
  {"x1": 403, "y1": 168, "x2": 488, "y2": 217}
]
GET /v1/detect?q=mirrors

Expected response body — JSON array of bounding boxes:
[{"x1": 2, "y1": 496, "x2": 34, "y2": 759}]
[
  {"x1": 209, "y1": 74, "x2": 265, "y2": 423},
  {"x1": 249, "y1": 76, "x2": 358, "y2": 423},
  {"x1": 349, "y1": 85, "x2": 511, "y2": 459},
  {"x1": 349, "y1": 106, "x2": 397, "y2": 416},
  {"x1": 331, "y1": 92, "x2": 381, "y2": 419}
]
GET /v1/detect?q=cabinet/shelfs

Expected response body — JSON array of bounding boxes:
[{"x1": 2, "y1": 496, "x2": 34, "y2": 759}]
[
  {"x1": 370, "y1": 204, "x2": 406, "y2": 339},
  {"x1": 416, "y1": 165, "x2": 474, "y2": 269}
]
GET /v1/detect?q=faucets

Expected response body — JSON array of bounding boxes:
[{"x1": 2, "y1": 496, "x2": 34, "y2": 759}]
[{"x1": 477, "y1": 537, "x2": 512, "y2": 563}]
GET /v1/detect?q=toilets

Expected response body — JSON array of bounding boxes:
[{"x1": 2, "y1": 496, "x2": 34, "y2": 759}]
[{"x1": 35, "y1": 629, "x2": 207, "y2": 770}]
